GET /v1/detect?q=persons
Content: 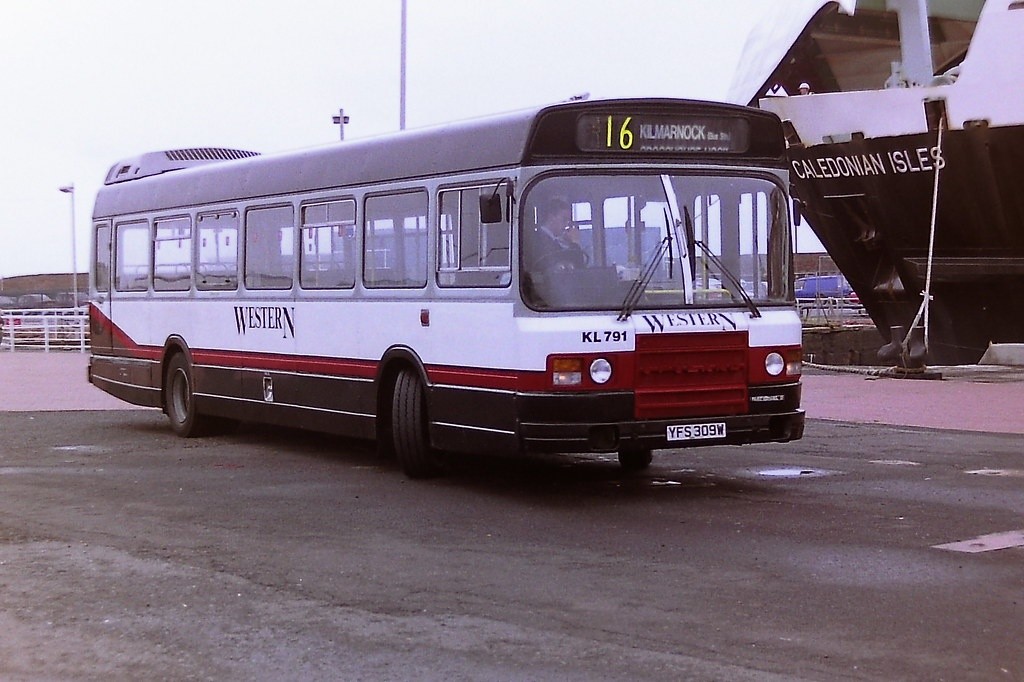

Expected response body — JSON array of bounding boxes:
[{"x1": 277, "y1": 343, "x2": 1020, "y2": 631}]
[
  {"x1": 529, "y1": 199, "x2": 584, "y2": 271},
  {"x1": 0, "y1": 317, "x2": 4, "y2": 345},
  {"x1": 799, "y1": 83, "x2": 814, "y2": 95}
]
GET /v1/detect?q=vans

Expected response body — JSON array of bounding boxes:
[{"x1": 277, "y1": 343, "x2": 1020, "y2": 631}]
[
  {"x1": 794, "y1": 276, "x2": 850, "y2": 298},
  {"x1": 56, "y1": 292, "x2": 89, "y2": 309},
  {"x1": 17, "y1": 295, "x2": 52, "y2": 309}
]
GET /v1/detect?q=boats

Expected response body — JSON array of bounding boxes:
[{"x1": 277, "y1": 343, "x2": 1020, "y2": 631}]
[{"x1": 731, "y1": 0, "x2": 1024, "y2": 380}]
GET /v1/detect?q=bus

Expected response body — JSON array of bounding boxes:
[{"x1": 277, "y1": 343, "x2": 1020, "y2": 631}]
[{"x1": 86, "y1": 99, "x2": 808, "y2": 477}]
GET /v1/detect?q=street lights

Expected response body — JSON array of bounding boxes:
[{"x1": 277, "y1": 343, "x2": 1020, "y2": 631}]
[
  {"x1": 59, "y1": 182, "x2": 80, "y2": 317},
  {"x1": 332, "y1": 108, "x2": 350, "y2": 141}
]
